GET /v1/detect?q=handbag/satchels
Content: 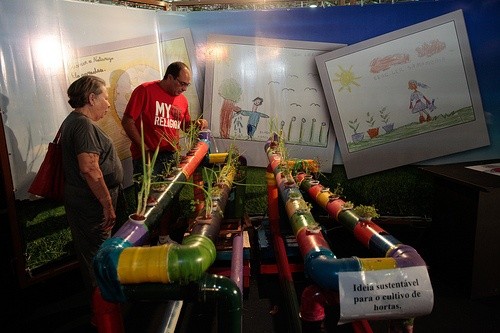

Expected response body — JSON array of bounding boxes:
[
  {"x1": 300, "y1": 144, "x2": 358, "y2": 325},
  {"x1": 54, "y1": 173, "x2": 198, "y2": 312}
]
[{"x1": 27, "y1": 118, "x2": 67, "y2": 202}]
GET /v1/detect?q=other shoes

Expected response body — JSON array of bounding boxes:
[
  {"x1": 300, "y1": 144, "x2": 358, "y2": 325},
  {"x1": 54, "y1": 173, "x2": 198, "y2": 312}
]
[{"x1": 157, "y1": 238, "x2": 182, "y2": 246}]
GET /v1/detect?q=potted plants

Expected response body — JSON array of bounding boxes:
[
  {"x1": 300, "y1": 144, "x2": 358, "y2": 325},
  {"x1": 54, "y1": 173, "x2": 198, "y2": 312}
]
[{"x1": 129, "y1": 103, "x2": 380, "y2": 234}]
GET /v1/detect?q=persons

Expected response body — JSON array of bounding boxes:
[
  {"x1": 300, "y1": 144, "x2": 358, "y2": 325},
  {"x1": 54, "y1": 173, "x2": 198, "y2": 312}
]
[
  {"x1": 121, "y1": 61, "x2": 210, "y2": 248},
  {"x1": 54, "y1": 75, "x2": 127, "y2": 328}
]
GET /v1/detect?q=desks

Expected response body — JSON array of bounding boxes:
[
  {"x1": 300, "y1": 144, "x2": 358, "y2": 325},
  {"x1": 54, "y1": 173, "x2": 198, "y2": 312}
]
[{"x1": 417, "y1": 159, "x2": 500, "y2": 301}]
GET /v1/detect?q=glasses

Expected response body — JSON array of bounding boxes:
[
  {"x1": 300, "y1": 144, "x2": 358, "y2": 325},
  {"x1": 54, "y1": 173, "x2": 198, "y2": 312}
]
[{"x1": 174, "y1": 77, "x2": 191, "y2": 87}]
